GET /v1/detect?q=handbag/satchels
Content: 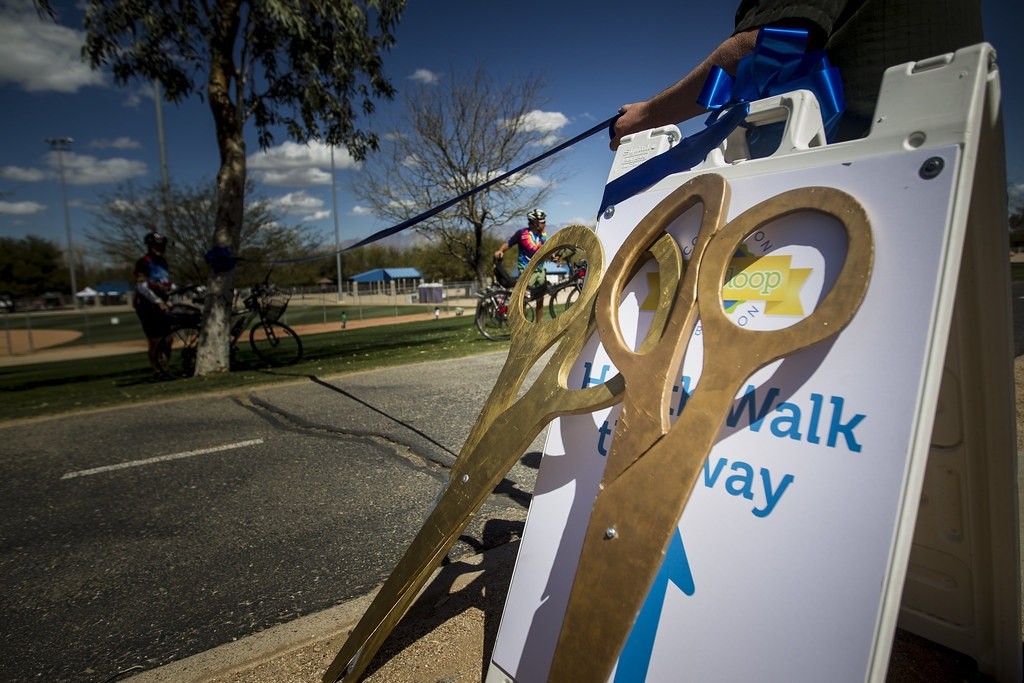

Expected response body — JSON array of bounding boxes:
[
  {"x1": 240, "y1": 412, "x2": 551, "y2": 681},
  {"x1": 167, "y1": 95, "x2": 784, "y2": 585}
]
[{"x1": 493, "y1": 264, "x2": 515, "y2": 287}]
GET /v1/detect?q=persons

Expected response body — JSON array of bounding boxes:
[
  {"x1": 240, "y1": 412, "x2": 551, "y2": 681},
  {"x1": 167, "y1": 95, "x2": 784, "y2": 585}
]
[
  {"x1": 494, "y1": 206, "x2": 562, "y2": 324},
  {"x1": 132, "y1": 231, "x2": 179, "y2": 379},
  {"x1": 434, "y1": 306, "x2": 441, "y2": 320},
  {"x1": 606, "y1": 0, "x2": 992, "y2": 168},
  {"x1": 340, "y1": 310, "x2": 347, "y2": 330}
]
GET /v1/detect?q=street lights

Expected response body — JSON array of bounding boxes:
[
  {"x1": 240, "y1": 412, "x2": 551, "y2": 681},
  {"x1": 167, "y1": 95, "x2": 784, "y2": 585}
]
[
  {"x1": 134, "y1": 38, "x2": 187, "y2": 263},
  {"x1": 45, "y1": 136, "x2": 79, "y2": 312},
  {"x1": 319, "y1": 111, "x2": 342, "y2": 297}
]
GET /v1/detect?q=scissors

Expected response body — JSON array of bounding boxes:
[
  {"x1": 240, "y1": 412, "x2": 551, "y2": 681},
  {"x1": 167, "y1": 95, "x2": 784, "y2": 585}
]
[
  {"x1": 321, "y1": 225, "x2": 685, "y2": 683},
  {"x1": 546, "y1": 174, "x2": 876, "y2": 683}
]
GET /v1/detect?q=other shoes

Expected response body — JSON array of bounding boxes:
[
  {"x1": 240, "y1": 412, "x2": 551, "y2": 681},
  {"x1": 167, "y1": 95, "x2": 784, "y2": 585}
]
[{"x1": 153, "y1": 368, "x2": 176, "y2": 380}]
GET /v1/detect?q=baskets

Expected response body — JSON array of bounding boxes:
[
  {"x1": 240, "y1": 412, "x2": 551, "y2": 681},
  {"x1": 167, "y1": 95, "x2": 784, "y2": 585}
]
[{"x1": 264, "y1": 271, "x2": 291, "y2": 318}]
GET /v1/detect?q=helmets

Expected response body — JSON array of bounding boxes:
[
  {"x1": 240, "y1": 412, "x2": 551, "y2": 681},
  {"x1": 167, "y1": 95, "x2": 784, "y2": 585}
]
[
  {"x1": 145, "y1": 233, "x2": 167, "y2": 244},
  {"x1": 527, "y1": 209, "x2": 549, "y2": 222}
]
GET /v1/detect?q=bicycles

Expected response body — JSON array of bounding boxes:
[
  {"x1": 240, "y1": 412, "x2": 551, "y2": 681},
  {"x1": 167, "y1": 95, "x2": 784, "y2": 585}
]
[
  {"x1": 473, "y1": 246, "x2": 586, "y2": 343},
  {"x1": 564, "y1": 259, "x2": 587, "y2": 313},
  {"x1": 166, "y1": 269, "x2": 306, "y2": 366},
  {"x1": 476, "y1": 271, "x2": 510, "y2": 328}
]
